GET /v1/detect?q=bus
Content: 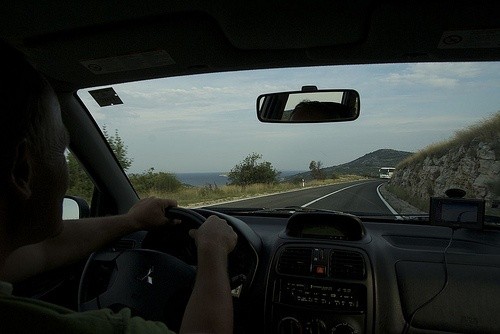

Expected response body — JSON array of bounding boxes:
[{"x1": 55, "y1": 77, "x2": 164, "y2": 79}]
[{"x1": 379, "y1": 166, "x2": 396, "y2": 178}]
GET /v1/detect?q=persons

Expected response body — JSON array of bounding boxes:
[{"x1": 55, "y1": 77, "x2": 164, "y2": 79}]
[{"x1": 0, "y1": 70, "x2": 237, "y2": 333}]
